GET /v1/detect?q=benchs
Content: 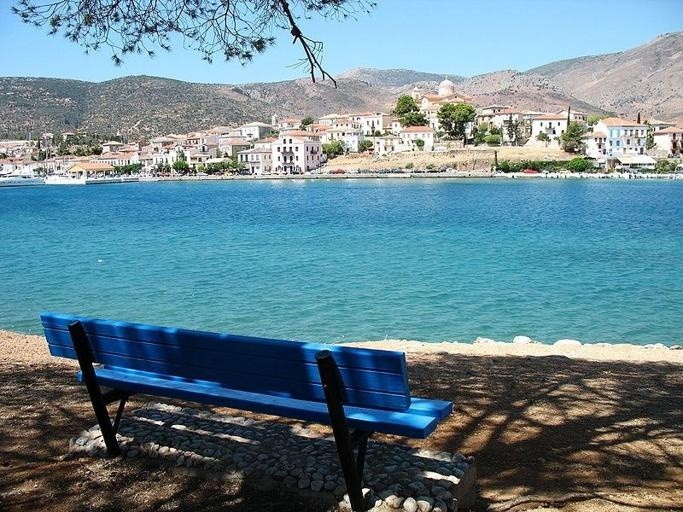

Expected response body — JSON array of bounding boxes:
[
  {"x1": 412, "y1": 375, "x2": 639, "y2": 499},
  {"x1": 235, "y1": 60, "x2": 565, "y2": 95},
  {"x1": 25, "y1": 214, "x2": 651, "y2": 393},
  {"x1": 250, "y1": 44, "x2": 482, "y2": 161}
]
[{"x1": 40, "y1": 313, "x2": 453, "y2": 512}]
